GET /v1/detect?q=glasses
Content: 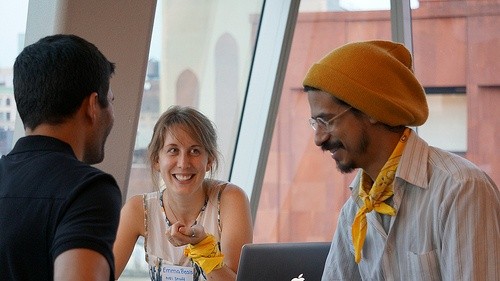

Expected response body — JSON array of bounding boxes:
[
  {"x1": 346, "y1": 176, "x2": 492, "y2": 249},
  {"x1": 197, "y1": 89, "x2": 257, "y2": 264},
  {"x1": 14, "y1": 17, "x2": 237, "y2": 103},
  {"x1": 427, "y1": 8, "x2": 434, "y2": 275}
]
[{"x1": 308, "y1": 107, "x2": 351, "y2": 131}]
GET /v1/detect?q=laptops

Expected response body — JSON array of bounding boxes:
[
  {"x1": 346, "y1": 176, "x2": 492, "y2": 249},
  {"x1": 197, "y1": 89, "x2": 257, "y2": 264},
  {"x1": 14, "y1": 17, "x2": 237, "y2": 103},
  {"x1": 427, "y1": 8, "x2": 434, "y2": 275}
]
[{"x1": 236, "y1": 242, "x2": 331, "y2": 281}]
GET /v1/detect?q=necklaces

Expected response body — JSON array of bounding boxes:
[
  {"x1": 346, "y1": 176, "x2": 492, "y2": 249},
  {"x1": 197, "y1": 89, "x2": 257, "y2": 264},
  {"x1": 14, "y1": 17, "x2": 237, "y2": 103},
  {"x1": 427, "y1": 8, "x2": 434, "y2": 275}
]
[{"x1": 168, "y1": 202, "x2": 178, "y2": 221}]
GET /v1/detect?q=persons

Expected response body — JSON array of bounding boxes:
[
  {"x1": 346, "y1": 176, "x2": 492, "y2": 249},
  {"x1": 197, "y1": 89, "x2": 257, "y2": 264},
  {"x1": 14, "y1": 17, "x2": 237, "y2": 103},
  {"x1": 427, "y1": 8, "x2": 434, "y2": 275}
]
[
  {"x1": 0, "y1": 34, "x2": 122, "y2": 281},
  {"x1": 302, "y1": 40, "x2": 500, "y2": 281},
  {"x1": 113, "y1": 106, "x2": 253, "y2": 281}
]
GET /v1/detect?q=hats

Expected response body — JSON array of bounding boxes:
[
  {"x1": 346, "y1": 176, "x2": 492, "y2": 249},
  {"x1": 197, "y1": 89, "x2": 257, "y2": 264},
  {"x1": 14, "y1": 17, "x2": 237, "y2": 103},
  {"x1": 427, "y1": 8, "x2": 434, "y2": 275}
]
[{"x1": 302, "y1": 39, "x2": 429, "y2": 127}]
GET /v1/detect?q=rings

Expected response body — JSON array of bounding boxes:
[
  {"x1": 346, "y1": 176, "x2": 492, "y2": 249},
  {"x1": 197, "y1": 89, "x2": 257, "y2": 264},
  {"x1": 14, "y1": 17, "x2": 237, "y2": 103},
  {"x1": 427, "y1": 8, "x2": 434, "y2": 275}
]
[{"x1": 191, "y1": 227, "x2": 196, "y2": 237}]
